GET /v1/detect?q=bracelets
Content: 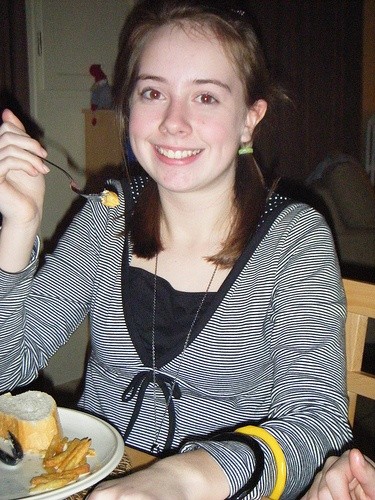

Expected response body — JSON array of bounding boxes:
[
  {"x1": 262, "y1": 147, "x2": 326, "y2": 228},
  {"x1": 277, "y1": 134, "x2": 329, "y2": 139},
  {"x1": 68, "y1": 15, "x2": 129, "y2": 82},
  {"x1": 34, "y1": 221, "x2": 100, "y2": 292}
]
[
  {"x1": 234, "y1": 424, "x2": 287, "y2": 500},
  {"x1": 209, "y1": 431, "x2": 265, "y2": 500}
]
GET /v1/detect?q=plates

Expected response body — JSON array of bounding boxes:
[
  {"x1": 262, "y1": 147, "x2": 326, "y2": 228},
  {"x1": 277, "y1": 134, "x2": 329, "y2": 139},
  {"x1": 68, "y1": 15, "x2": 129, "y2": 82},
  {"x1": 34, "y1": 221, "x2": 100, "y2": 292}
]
[{"x1": 0, "y1": 407, "x2": 124, "y2": 500}]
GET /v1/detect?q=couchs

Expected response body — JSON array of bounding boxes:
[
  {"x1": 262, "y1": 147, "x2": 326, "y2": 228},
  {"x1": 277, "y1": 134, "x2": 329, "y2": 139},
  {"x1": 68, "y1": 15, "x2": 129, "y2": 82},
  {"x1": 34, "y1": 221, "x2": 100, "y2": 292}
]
[{"x1": 305, "y1": 152, "x2": 375, "y2": 266}]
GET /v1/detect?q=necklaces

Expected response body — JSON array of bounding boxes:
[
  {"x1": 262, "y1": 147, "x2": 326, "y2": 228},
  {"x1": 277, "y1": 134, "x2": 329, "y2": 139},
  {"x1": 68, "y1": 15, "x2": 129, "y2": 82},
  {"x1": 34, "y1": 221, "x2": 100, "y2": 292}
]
[{"x1": 150, "y1": 205, "x2": 240, "y2": 457}]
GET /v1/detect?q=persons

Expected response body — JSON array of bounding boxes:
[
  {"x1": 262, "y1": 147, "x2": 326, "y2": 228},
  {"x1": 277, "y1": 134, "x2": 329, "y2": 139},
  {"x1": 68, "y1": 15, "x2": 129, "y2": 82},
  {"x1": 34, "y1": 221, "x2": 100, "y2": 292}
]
[{"x1": 0, "y1": 0, "x2": 354, "y2": 500}]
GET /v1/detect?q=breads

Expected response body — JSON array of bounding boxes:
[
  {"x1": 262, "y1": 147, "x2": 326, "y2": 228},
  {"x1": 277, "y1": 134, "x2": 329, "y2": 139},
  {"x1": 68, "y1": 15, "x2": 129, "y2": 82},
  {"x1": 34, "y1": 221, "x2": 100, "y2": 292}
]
[{"x1": 0, "y1": 390, "x2": 62, "y2": 454}]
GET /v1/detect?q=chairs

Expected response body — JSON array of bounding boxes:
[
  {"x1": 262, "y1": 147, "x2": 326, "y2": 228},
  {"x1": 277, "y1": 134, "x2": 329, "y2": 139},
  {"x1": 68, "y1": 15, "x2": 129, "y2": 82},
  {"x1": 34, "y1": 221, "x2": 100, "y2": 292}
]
[{"x1": 343, "y1": 279, "x2": 375, "y2": 430}]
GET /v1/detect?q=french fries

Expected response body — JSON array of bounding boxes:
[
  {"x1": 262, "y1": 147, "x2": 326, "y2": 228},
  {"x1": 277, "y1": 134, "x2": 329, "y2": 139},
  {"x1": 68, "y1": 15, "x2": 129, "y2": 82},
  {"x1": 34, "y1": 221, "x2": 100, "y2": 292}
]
[{"x1": 29, "y1": 435, "x2": 96, "y2": 494}]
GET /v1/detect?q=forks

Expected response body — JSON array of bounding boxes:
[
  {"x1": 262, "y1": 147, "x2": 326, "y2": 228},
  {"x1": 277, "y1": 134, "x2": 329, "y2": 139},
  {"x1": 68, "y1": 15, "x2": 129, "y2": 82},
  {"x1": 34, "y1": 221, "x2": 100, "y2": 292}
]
[{"x1": 33, "y1": 156, "x2": 119, "y2": 206}]
